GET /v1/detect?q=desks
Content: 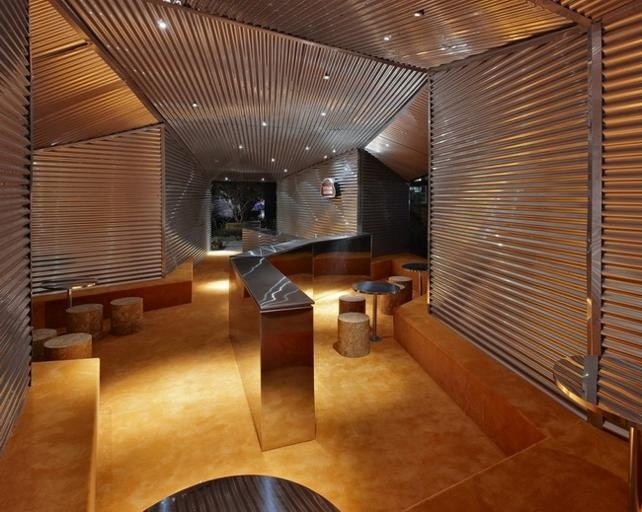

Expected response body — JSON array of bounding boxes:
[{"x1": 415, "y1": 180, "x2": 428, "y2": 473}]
[
  {"x1": 550, "y1": 351, "x2": 642, "y2": 512},
  {"x1": 402, "y1": 262, "x2": 429, "y2": 296},
  {"x1": 41, "y1": 277, "x2": 98, "y2": 311}
]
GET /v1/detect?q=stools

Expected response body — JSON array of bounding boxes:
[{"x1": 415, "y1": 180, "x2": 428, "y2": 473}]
[
  {"x1": 31, "y1": 297, "x2": 145, "y2": 362},
  {"x1": 336, "y1": 275, "x2": 413, "y2": 359}
]
